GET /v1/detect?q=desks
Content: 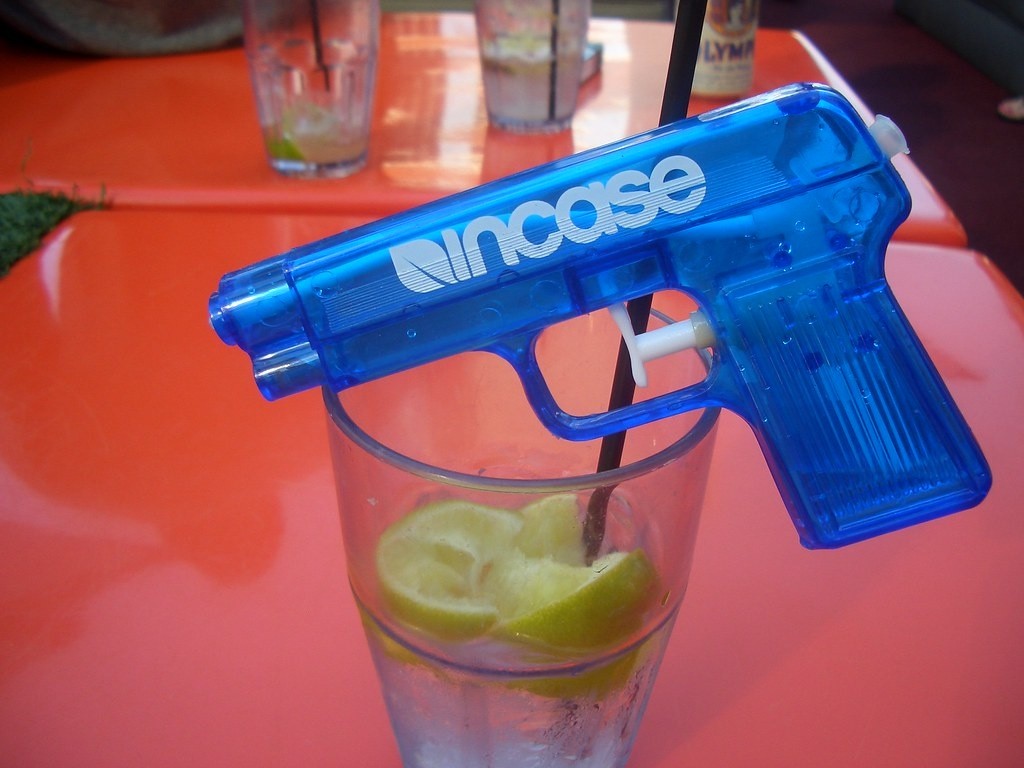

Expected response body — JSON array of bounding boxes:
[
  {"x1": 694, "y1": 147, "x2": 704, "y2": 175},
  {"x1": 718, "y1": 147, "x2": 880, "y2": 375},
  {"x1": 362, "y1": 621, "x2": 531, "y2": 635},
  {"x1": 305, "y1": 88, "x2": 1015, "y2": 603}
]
[
  {"x1": 0, "y1": 11, "x2": 971, "y2": 248},
  {"x1": 0, "y1": 207, "x2": 1024, "y2": 768}
]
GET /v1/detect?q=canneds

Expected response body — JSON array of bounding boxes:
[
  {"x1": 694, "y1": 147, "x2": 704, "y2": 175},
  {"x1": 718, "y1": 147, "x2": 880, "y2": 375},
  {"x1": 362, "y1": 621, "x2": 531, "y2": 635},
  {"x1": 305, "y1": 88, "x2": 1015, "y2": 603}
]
[{"x1": 673, "y1": 0, "x2": 758, "y2": 97}]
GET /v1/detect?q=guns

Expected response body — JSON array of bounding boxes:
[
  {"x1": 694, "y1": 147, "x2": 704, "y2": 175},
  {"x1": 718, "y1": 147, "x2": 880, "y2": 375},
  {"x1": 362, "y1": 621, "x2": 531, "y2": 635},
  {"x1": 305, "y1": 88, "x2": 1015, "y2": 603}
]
[{"x1": 203, "y1": 81, "x2": 995, "y2": 552}]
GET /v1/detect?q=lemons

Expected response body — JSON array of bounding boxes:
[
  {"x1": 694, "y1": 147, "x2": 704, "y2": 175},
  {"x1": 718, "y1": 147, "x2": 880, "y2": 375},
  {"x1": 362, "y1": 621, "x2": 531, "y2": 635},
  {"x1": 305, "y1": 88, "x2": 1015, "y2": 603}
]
[
  {"x1": 268, "y1": 113, "x2": 309, "y2": 161},
  {"x1": 375, "y1": 493, "x2": 656, "y2": 696}
]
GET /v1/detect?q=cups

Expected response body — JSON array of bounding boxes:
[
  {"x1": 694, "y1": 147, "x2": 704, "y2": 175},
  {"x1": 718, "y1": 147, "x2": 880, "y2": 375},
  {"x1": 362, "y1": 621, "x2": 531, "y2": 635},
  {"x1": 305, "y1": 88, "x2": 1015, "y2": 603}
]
[
  {"x1": 476, "y1": 0, "x2": 593, "y2": 136},
  {"x1": 322, "y1": 303, "x2": 721, "y2": 768},
  {"x1": 243, "y1": 0, "x2": 379, "y2": 180},
  {"x1": 674, "y1": 0, "x2": 758, "y2": 99}
]
